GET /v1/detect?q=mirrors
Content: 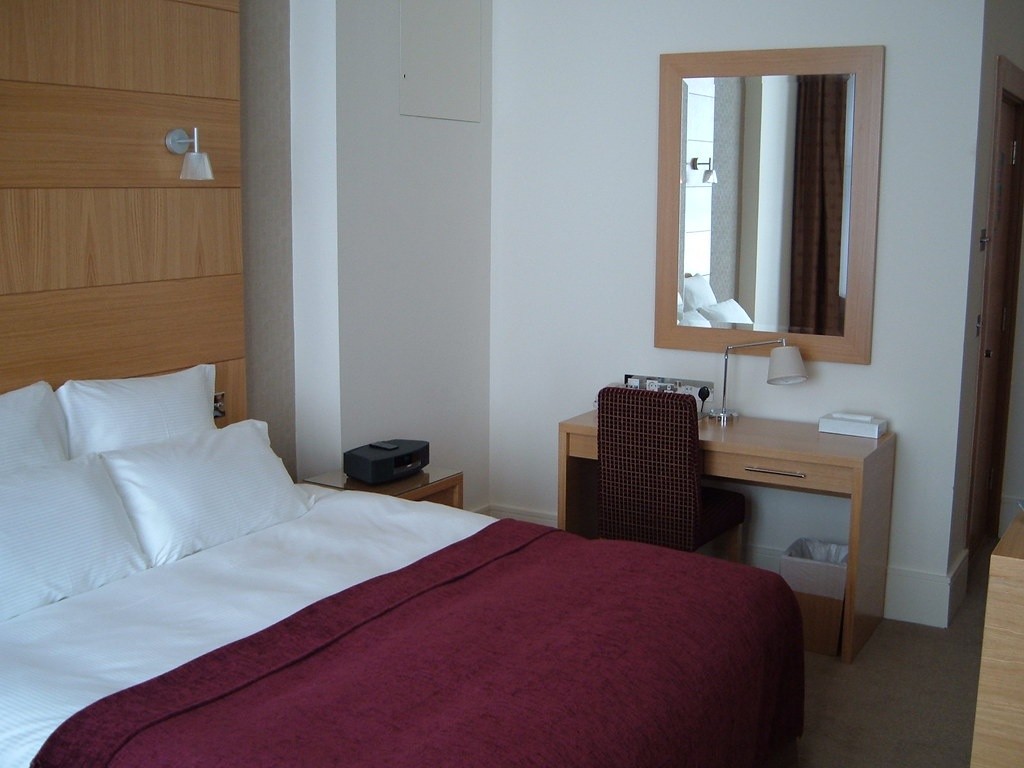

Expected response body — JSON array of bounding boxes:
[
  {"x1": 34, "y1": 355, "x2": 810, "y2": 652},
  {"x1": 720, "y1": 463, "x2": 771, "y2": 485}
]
[{"x1": 654, "y1": 45, "x2": 885, "y2": 365}]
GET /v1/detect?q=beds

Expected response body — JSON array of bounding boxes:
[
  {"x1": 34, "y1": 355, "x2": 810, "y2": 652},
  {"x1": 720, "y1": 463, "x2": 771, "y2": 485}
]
[{"x1": 0, "y1": 0, "x2": 811, "y2": 768}]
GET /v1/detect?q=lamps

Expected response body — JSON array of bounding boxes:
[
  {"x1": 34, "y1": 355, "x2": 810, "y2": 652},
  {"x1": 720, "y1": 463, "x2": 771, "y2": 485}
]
[
  {"x1": 165, "y1": 128, "x2": 215, "y2": 181},
  {"x1": 691, "y1": 157, "x2": 718, "y2": 184},
  {"x1": 708, "y1": 337, "x2": 809, "y2": 422}
]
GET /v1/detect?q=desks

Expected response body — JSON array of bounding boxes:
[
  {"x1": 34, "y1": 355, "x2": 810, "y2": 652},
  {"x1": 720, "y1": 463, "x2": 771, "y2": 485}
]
[{"x1": 557, "y1": 411, "x2": 896, "y2": 665}]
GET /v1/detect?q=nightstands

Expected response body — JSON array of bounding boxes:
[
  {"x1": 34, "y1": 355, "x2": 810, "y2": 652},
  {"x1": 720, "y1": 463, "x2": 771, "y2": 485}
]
[{"x1": 302, "y1": 465, "x2": 464, "y2": 509}]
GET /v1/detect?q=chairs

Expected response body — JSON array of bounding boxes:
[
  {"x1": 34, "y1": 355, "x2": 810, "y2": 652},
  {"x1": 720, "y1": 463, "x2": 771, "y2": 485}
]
[{"x1": 597, "y1": 387, "x2": 747, "y2": 562}]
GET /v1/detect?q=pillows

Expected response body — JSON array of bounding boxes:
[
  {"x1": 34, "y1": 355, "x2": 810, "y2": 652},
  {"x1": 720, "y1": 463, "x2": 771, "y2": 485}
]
[
  {"x1": 697, "y1": 299, "x2": 754, "y2": 324},
  {"x1": 0, "y1": 451, "x2": 149, "y2": 624},
  {"x1": 684, "y1": 273, "x2": 717, "y2": 309},
  {"x1": 52, "y1": 363, "x2": 217, "y2": 457},
  {"x1": 678, "y1": 310, "x2": 712, "y2": 328},
  {"x1": 99, "y1": 419, "x2": 317, "y2": 568},
  {"x1": 0, "y1": 380, "x2": 68, "y2": 473}
]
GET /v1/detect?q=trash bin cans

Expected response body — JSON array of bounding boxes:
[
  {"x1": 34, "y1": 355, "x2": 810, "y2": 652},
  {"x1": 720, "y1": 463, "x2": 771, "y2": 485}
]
[{"x1": 779, "y1": 537, "x2": 850, "y2": 656}]
